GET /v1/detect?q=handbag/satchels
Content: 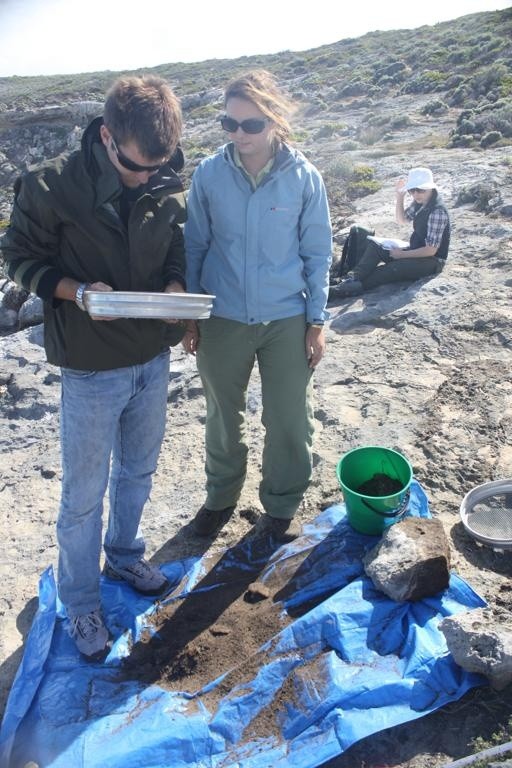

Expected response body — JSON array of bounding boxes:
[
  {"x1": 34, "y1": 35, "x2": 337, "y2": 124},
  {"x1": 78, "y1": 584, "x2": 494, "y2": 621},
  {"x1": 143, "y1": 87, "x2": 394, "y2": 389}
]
[{"x1": 346, "y1": 226, "x2": 375, "y2": 273}]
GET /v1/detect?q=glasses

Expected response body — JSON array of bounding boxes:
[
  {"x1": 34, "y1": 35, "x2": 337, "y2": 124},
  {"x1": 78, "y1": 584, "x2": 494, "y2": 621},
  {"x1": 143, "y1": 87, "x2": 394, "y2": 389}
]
[
  {"x1": 221, "y1": 113, "x2": 268, "y2": 135},
  {"x1": 110, "y1": 137, "x2": 162, "y2": 173},
  {"x1": 408, "y1": 188, "x2": 426, "y2": 194}
]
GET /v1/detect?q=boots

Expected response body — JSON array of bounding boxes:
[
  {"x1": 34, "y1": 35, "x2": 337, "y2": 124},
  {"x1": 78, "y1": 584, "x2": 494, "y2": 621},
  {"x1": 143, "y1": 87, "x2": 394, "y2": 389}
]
[
  {"x1": 331, "y1": 245, "x2": 381, "y2": 283},
  {"x1": 328, "y1": 279, "x2": 366, "y2": 301}
]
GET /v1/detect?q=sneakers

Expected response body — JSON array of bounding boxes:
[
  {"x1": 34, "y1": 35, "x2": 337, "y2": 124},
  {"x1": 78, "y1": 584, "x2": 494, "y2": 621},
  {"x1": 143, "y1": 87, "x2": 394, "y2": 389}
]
[
  {"x1": 272, "y1": 517, "x2": 301, "y2": 542},
  {"x1": 102, "y1": 559, "x2": 170, "y2": 596},
  {"x1": 193, "y1": 505, "x2": 225, "y2": 538},
  {"x1": 70, "y1": 611, "x2": 112, "y2": 663}
]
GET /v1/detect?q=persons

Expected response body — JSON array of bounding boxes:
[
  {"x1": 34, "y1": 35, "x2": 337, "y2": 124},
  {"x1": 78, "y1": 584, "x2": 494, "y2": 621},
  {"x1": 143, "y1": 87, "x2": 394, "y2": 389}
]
[
  {"x1": 327, "y1": 167, "x2": 452, "y2": 300},
  {"x1": 181, "y1": 68, "x2": 333, "y2": 536},
  {"x1": 1, "y1": 72, "x2": 190, "y2": 663}
]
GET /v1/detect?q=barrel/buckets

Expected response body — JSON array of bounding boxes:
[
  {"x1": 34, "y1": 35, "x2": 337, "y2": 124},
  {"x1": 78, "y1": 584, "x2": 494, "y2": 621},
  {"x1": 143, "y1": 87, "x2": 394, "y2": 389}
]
[{"x1": 337, "y1": 445, "x2": 413, "y2": 536}]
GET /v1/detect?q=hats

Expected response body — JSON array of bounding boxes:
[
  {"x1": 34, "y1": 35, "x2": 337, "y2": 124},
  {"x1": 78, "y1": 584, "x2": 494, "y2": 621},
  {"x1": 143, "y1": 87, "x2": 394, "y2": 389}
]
[{"x1": 399, "y1": 168, "x2": 436, "y2": 192}]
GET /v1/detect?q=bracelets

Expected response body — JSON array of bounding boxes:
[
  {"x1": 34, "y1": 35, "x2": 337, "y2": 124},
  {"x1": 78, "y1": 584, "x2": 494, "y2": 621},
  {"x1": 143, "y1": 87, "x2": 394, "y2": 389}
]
[
  {"x1": 307, "y1": 324, "x2": 323, "y2": 329},
  {"x1": 75, "y1": 282, "x2": 91, "y2": 313}
]
